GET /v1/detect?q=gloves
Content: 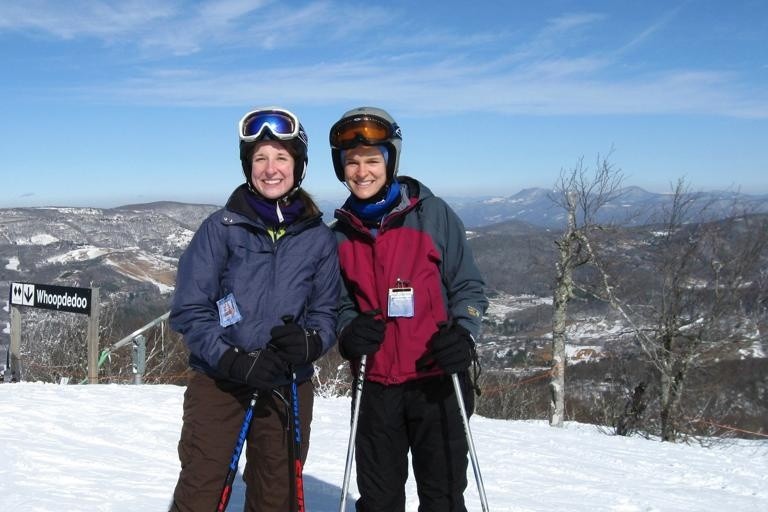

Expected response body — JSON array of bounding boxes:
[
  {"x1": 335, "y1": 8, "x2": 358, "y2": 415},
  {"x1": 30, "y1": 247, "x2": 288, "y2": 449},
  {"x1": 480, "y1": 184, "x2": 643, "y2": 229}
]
[
  {"x1": 427, "y1": 317, "x2": 479, "y2": 375},
  {"x1": 336, "y1": 307, "x2": 386, "y2": 361},
  {"x1": 269, "y1": 315, "x2": 324, "y2": 364},
  {"x1": 216, "y1": 341, "x2": 279, "y2": 387}
]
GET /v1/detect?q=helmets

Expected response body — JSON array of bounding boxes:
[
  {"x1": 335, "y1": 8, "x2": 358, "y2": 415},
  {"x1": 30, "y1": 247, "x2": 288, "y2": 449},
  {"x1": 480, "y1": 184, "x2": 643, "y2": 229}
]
[
  {"x1": 328, "y1": 105, "x2": 404, "y2": 199},
  {"x1": 237, "y1": 107, "x2": 309, "y2": 202}
]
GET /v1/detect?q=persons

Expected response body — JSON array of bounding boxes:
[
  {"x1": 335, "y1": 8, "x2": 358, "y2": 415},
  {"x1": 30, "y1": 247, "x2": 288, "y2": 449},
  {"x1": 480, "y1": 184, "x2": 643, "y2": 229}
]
[
  {"x1": 326, "y1": 106, "x2": 490, "y2": 512},
  {"x1": 167, "y1": 106, "x2": 341, "y2": 512}
]
